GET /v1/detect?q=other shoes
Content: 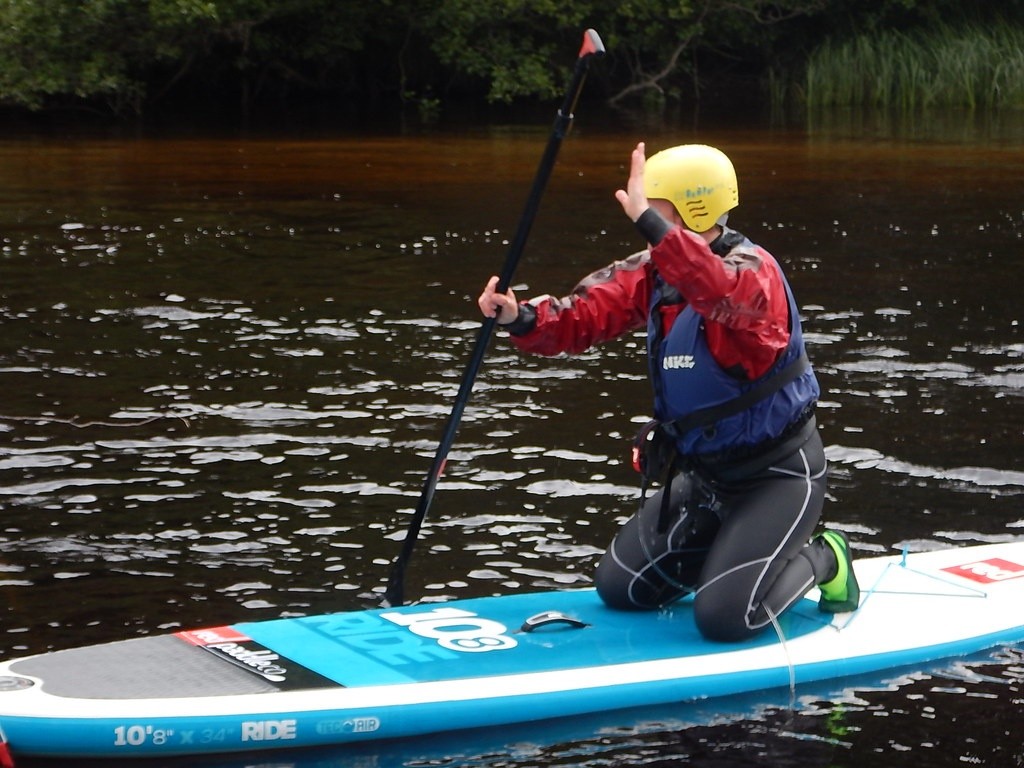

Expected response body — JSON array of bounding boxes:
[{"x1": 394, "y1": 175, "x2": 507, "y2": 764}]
[{"x1": 813, "y1": 528, "x2": 859, "y2": 615}]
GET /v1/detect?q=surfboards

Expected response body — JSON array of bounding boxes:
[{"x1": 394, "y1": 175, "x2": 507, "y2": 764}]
[{"x1": 0, "y1": 539, "x2": 1024, "y2": 759}]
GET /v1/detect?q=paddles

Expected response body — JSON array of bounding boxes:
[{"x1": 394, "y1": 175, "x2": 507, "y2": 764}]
[{"x1": 380, "y1": 26, "x2": 606, "y2": 611}]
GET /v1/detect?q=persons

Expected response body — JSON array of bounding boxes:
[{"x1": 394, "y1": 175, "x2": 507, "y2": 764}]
[{"x1": 477, "y1": 142, "x2": 861, "y2": 641}]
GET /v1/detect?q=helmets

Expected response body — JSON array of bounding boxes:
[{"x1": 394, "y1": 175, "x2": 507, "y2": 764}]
[{"x1": 641, "y1": 143, "x2": 739, "y2": 232}]
